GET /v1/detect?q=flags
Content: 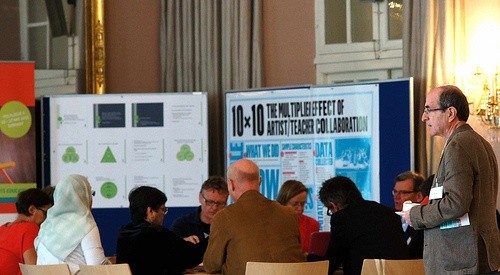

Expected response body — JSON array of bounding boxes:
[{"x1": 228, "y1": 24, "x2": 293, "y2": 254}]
[{"x1": 0, "y1": 59, "x2": 36, "y2": 107}]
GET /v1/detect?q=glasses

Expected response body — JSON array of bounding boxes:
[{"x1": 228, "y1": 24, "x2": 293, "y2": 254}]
[
  {"x1": 89, "y1": 191, "x2": 96, "y2": 197},
  {"x1": 392, "y1": 189, "x2": 414, "y2": 196},
  {"x1": 327, "y1": 206, "x2": 333, "y2": 216},
  {"x1": 157, "y1": 207, "x2": 168, "y2": 215},
  {"x1": 288, "y1": 201, "x2": 305, "y2": 207},
  {"x1": 201, "y1": 194, "x2": 227, "y2": 209},
  {"x1": 423, "y1": 108, "x2": 445, "y2": 114}
]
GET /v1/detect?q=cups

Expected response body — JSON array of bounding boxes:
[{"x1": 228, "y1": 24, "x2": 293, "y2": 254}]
[{"x1": 402, "y1": 202, "x2": 421, "y2": 211}]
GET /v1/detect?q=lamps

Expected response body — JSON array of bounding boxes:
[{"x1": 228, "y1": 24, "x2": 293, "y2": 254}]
[{"x1": 460, "y1": 64, "x2": 500, "y2": 129}]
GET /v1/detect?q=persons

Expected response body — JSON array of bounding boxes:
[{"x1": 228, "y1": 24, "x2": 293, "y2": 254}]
[
  {"x1": 203, "y1": 158, "x2": 307, "y2": 275},
  {"x1": 389, "y1": 172, "x2": 428, "y2": 260},
  {"x1": 402, "y1": 84, "x2": 499, "y2": 275},
  {"x1": 170, "y1": 176, "x2": 229, "y2": 245},
  {"x1": 276, "y1": 180, "x2": 321, "y2": 262},
  {"x1": 33, "y1": 174, "x2": 116, "y2": 266},
  {"x1": 116, "y1": 185, "x2": 207, "y2": 275},
  {"x1": 0, "y1": 188, "x2": 52, "y2": 275},
  {"x1": 303, "y1": 176, "x2": 403, "y2": 275}
]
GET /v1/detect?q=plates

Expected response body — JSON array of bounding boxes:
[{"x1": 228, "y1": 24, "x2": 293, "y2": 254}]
[{"x1": 395, "y1": 212, "x2": 405, "y2": 216}]
